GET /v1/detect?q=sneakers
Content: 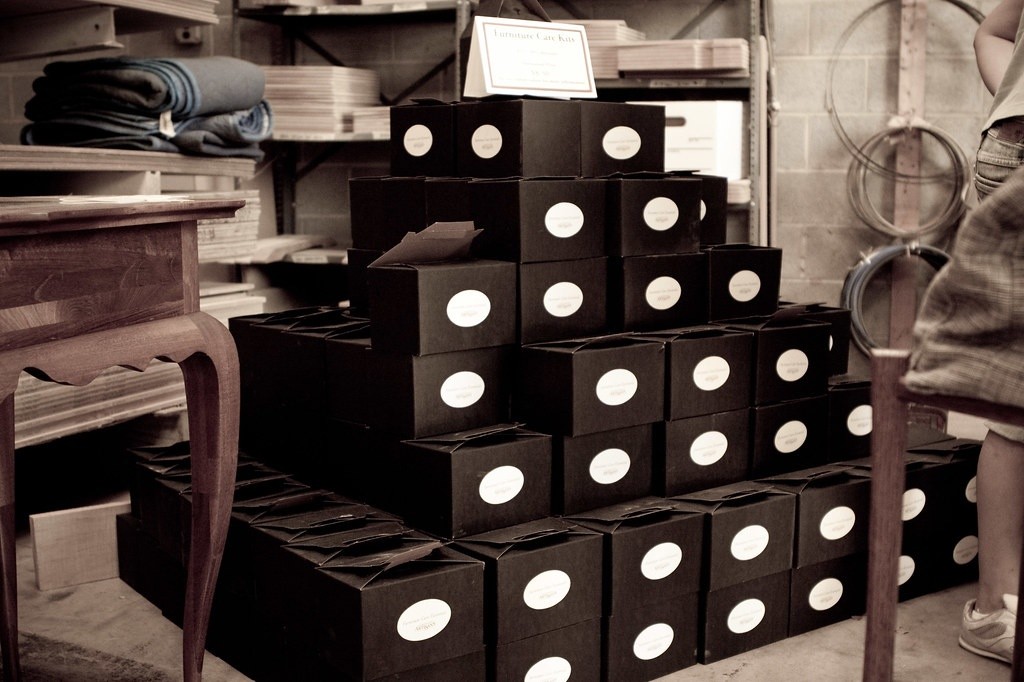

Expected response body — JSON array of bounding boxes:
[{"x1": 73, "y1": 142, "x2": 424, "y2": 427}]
[{"x1": 958, "y1": 593, "x2": 1018, "y2": 663}]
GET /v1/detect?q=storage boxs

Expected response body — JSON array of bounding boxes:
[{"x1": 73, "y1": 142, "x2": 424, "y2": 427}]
[{"x1": 114, "y1": 101, "x2": 985, "y2": 682}]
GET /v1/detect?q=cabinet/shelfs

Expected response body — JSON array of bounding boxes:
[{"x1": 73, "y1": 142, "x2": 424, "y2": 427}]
[{"x1": 0, "y1": 0, "x2": 781, "y2": 451}]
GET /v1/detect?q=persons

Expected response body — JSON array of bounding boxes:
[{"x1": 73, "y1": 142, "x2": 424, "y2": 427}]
[{"x1": 957, "y1": 0, "x2": 1024, "y2": 663}]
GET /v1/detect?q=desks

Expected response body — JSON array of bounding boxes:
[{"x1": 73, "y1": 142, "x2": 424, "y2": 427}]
[{"x1": 0, "y1": 195, "x2": 246, "y2": 682}]
